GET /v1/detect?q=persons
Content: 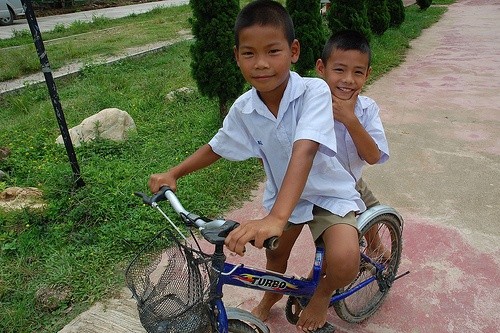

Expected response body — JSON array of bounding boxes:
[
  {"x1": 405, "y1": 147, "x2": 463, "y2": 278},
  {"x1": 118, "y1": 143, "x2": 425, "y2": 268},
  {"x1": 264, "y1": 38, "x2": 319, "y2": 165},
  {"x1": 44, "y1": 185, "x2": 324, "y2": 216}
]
[
  {"x1": 149, "y1": 0, "x2": 369, "y2": 332},
  {"x1": 307, "y1": 30, "x2": 395, "y2": 279}
]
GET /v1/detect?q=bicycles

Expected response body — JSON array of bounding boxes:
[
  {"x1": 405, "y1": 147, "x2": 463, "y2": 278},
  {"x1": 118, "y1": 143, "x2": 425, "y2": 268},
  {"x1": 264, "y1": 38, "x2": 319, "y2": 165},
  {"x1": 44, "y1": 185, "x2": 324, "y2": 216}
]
[{"x1": 124, "y1": 186, "x2": 410, "y2": 333}]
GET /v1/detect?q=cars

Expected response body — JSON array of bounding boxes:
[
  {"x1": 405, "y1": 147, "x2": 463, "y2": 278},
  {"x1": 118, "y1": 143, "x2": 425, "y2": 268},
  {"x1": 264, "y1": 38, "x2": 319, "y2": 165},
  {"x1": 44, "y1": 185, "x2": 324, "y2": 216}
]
[{"x1": 0, "y1": 0, "x2": 25, "y2": 26}]
